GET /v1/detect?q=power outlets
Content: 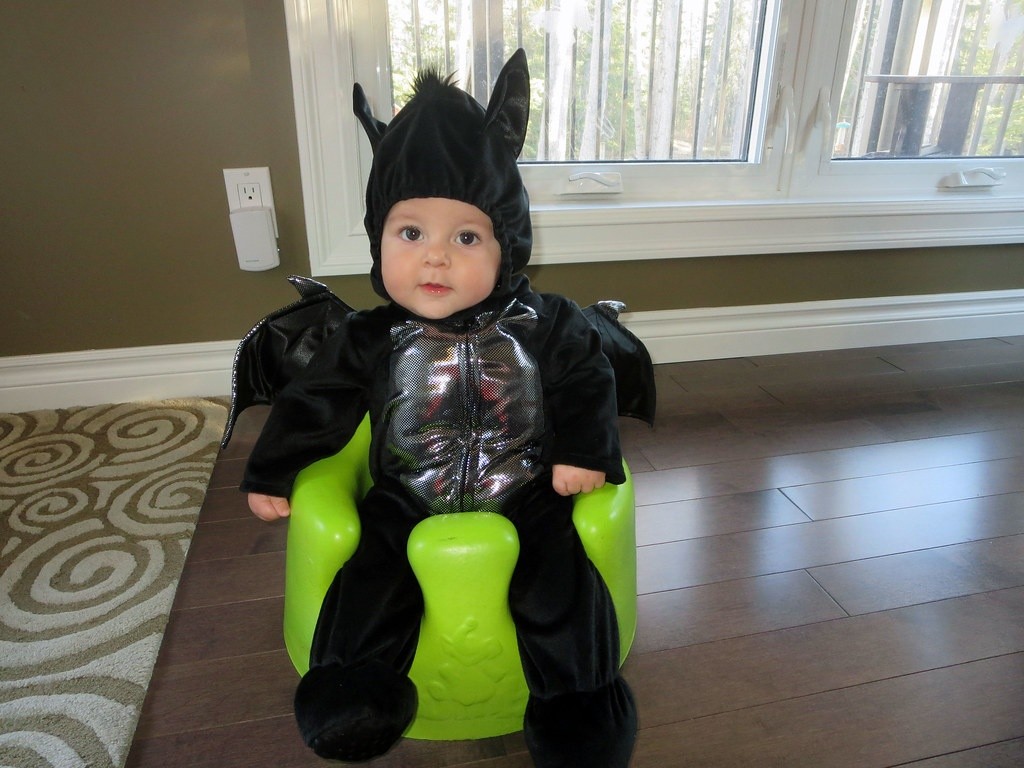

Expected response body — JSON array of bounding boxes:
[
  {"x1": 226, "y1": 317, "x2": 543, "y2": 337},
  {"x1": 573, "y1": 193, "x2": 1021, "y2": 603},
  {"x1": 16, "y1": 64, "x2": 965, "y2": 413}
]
[{"x1": 223, "y1": 166, "x2": 281, "y2": 241}]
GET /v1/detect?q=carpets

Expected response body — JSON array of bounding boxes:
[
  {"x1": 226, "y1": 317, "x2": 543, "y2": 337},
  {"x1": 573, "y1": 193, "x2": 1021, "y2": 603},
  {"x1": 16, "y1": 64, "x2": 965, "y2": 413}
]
[{"x1": 0, "y1": 392, "x2": 240, "y2": 765}]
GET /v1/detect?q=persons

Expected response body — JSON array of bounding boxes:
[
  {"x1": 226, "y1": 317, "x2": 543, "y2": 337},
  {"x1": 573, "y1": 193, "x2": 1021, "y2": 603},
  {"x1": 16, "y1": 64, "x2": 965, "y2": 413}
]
[{"x1": 239, "y1": 51, "x2": 641, "y2": 766}]
